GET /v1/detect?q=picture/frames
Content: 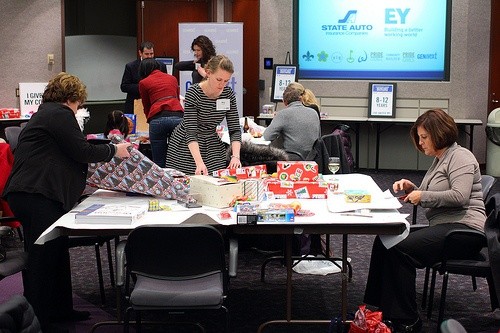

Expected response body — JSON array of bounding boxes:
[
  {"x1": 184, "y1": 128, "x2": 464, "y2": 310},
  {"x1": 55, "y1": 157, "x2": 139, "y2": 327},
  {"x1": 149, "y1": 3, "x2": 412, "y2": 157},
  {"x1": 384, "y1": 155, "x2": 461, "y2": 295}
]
[
  {"x1": 368, "y1": 82, "x2": 396, "y2": 118},
  {"x1": 270, "y1": 64, "x2": 299, "y2": 103}
]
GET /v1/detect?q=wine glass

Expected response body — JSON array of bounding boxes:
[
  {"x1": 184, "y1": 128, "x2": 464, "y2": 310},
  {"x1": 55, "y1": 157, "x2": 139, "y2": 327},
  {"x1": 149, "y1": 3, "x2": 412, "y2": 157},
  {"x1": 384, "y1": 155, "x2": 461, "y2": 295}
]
[{"x1": 328, "y1": 157, "x2": 340, "y2": 176}]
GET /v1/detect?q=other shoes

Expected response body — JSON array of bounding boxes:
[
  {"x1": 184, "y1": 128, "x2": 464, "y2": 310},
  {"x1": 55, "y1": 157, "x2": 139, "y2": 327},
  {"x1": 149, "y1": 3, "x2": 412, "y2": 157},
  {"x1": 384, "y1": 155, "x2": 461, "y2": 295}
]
[
  {"x1": 396, "y1": 316, "x2": 423, "y2": 333},
  {"x1": 73, "y1": 310, "x2": 91, "y2": 321}
]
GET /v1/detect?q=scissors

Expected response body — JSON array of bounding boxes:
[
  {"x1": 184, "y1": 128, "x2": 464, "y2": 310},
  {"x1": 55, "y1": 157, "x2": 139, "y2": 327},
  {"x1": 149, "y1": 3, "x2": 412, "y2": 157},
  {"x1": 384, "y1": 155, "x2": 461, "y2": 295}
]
[{"x1": 384, "y1": 195, "x2": 410, "y2": 203}]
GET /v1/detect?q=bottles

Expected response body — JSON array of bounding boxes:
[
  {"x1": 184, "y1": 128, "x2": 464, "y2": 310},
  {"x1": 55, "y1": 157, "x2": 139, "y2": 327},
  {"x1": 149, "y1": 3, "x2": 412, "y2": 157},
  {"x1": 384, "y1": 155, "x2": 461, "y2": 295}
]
[{"x1": 244, "y1": 118, "x2": 250, "y2": 133}]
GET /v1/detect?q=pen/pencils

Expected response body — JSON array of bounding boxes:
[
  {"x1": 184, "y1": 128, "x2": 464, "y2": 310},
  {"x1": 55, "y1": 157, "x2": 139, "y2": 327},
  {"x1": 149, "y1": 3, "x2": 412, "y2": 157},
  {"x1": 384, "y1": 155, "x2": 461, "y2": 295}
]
[{"x1": 342, "y1": 213, "x2": 373, "y2": 218}]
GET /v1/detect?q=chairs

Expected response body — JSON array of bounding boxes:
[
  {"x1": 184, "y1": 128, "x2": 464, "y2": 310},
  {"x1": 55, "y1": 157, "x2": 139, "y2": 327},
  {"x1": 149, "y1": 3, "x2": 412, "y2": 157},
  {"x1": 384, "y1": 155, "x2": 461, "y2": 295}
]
[
  {"x1": 284, "y1": 129, "x2": 353, "y2": 171},
  {"x1": 411, "y1": 174, "x2": 500, "y2": 328},
  {"x1": 121, "y1": 223, "x2": 229, "y2": 333}
]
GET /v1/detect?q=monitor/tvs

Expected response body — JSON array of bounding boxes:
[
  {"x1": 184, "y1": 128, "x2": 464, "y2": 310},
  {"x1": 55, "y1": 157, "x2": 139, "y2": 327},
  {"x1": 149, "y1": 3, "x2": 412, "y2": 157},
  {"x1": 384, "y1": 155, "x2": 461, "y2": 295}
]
[{"x1": 291, "y1": 0, "x2": 452, "y2": 82}]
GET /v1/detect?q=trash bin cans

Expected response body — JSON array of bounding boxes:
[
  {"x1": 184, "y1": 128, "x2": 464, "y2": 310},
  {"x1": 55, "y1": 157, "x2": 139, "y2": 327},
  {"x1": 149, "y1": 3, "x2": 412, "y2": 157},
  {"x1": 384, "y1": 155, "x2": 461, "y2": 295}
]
[{"x1": 485, "y1": 107, "x2": 500, "y2": 177}]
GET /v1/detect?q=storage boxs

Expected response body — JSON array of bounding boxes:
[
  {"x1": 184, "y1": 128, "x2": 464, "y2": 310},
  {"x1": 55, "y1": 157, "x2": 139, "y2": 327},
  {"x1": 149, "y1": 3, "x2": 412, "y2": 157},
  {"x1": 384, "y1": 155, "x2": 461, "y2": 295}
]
[
  {"x1": 0, "y1": 108, "x2": 20, "y2": 119},
  {"x1": 342, "y1": 189, "x2": 371, "y2": 203},
  {"x1": 189, "y1": 159, "x2": 340, "y2": 205}
]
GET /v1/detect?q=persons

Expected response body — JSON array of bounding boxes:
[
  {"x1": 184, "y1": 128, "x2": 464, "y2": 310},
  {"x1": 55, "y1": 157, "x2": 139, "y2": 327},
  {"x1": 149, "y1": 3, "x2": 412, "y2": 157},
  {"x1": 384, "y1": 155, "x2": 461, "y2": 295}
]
[
  {"x1": 263, "y1": 82, "x2": 322, "y2": 161},
  {"x1": 0, "y1": 72, "x2": 131, "y2": 333},
  {"x1": 364, "y1": 107, "x2": 486, "y2": 333},
  {"x1": 105, "y1": 34, "x2": 242, "y2": 176}
]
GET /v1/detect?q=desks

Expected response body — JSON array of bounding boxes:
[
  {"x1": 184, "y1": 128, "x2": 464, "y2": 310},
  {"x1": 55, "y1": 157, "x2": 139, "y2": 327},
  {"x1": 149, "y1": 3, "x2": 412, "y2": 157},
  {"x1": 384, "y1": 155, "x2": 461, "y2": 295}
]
[
  {"x1": 258, "y1": 113, "x2": 483, "y2": 173},
  {"x1": 0, "y1": 118, "x2": 31, "y2": 121},
  {"x1": 35, "y1": 173, "x2": 412, "y2": 333}
]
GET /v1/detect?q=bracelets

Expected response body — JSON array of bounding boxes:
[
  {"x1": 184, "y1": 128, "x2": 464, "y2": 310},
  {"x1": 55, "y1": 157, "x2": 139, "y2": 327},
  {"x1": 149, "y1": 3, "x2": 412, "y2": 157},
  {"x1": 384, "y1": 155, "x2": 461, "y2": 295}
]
[{"x1": 230, "y1": 155, "x2": 240, "y2": 160}]
